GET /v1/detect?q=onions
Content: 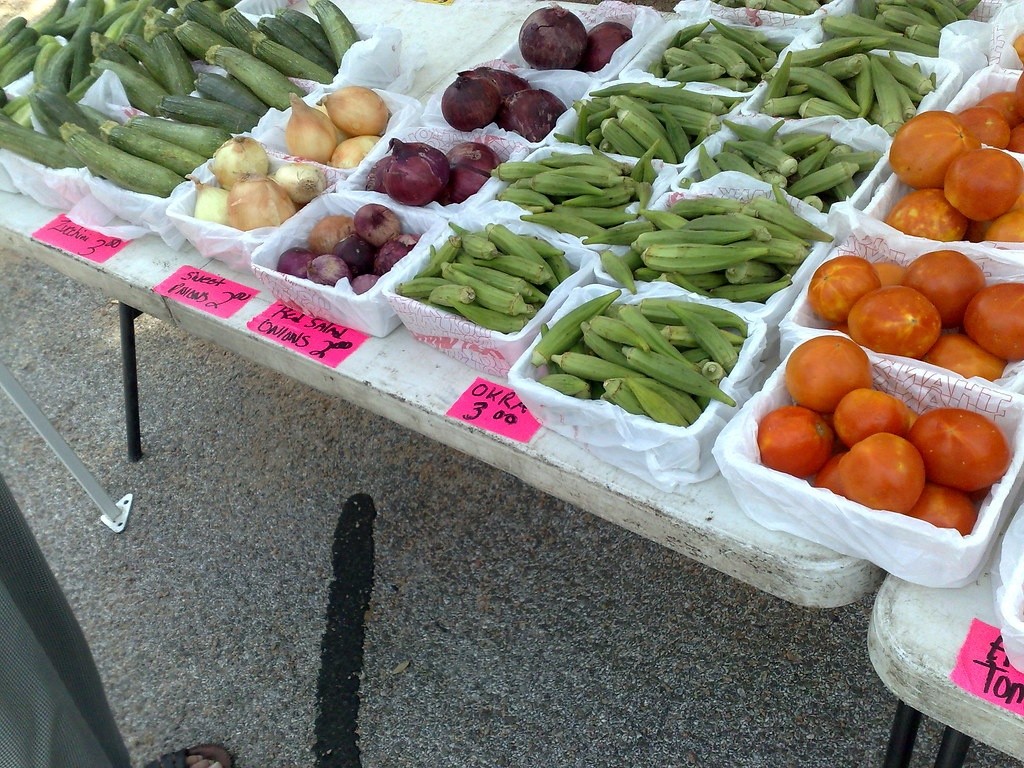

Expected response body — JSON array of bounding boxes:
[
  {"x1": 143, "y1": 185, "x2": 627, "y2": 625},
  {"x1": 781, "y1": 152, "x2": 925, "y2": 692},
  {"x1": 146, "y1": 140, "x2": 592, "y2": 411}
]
[{"x1": 182, "y1": 0, "x2": 634, "y2": 299}]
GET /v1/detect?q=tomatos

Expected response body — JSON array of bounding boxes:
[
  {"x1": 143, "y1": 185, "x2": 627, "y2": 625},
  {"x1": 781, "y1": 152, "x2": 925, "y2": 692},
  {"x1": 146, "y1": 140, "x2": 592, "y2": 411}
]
[{"x1": 758, "y1": 33, "x2": 1024, "y2": 536}]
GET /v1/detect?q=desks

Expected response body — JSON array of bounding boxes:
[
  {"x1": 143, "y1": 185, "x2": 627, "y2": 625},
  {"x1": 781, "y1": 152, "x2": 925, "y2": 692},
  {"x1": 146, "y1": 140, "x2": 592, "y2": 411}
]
[{"x1": 1, "y1": 193, "x2": 1024, "y2": 768}]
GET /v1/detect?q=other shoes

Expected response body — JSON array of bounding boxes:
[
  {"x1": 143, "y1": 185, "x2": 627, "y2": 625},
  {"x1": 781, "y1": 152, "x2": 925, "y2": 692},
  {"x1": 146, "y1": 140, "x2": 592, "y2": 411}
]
[{"x1": 146, "y1": 743, "x2": 233, "y2": 768}]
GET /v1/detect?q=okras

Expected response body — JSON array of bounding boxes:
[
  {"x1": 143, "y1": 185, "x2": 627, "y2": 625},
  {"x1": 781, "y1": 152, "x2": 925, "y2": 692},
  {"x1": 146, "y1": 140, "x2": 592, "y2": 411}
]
[{"x1": 395, "y1": 0, "x2": 985, "y2": 433}]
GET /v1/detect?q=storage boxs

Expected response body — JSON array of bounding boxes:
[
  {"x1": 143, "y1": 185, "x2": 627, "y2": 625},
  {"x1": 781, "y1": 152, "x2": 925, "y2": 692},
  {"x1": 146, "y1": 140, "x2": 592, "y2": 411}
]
[{"x1": 1, "y1": 1, "x2": 1024, "y2": 675}]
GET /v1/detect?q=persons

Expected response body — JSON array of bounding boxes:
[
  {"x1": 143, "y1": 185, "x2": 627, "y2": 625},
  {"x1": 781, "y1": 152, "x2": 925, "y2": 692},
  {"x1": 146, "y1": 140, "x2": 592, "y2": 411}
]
[{"x1": 1, "y1": 470, "x2": 235, "y2": 767}]
